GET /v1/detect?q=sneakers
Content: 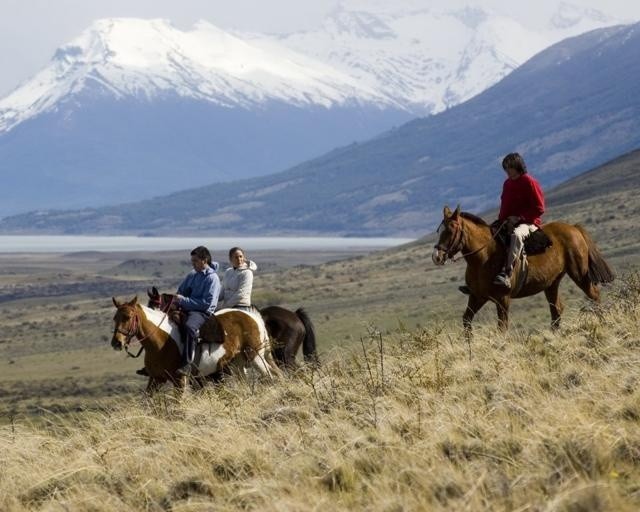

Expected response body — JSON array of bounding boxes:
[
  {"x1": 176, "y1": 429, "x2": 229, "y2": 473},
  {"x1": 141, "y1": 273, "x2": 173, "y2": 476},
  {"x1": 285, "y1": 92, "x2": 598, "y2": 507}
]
[
  {"x1": 458, "y1": 285, "x2": 469, "y2": 292},
  {"x1": 176, "y1": 365, "x2": 191, "y2": 377},
  {"x1": 135, "y1": 367, "x2": 146, "y2": 375},
  {"x1": 496, "y1": 274, "x2": 512, "y2": 286}
]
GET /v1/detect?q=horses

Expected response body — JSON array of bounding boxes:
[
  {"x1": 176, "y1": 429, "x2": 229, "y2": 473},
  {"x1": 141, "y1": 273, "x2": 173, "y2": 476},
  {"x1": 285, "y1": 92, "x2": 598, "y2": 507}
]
[
  {"x1": 146, "y1": 286, "x2": 321, "y2": 394},
  {"x1": 111, "y1": 294, "x2": 287, "y2": 409},
  {"x1": 431, "y1": 203, "x2": 615, "y2": 343}
]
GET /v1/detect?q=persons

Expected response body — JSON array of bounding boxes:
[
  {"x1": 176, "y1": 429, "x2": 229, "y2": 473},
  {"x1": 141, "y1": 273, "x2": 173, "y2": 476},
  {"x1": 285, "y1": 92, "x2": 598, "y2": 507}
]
[
  {"x1": 169, "y1": 246, "x2": 220, "y2": 377},
  {"x1": 219, "y1": 247, "x2": 258, "y2": 312},
  {"x1": 493, "y1": 151, "x2": 545, "y2": 286}
]
